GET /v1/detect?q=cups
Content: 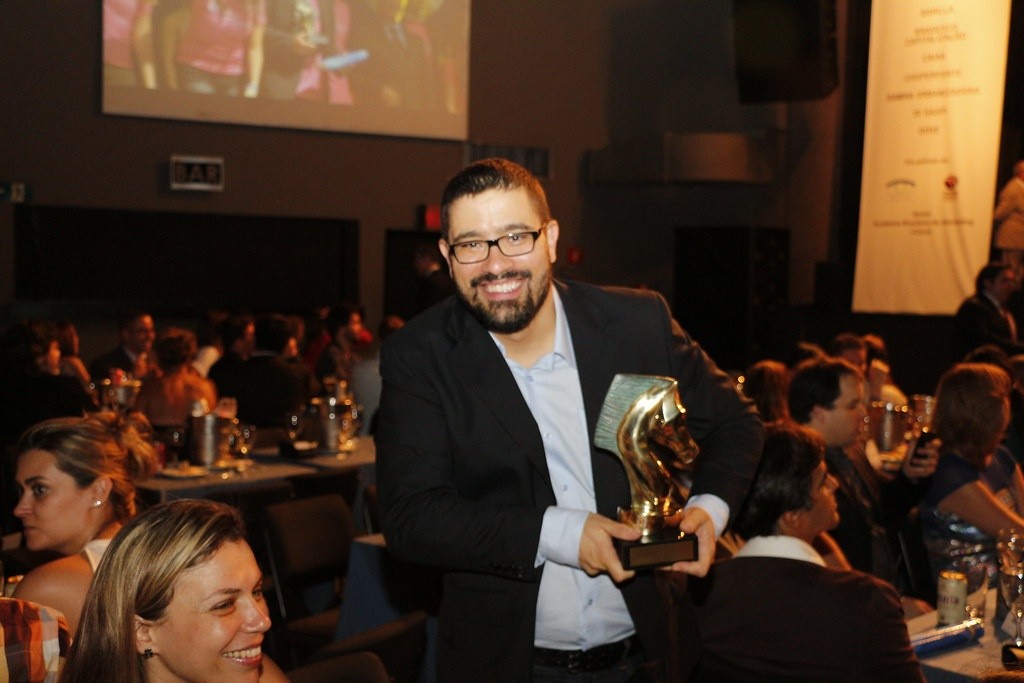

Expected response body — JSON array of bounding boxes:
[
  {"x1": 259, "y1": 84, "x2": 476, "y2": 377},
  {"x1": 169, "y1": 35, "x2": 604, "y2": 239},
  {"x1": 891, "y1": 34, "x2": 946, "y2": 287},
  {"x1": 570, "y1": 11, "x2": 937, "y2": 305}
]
[
  {"x1": 954, "y1": 559, "x2": 987, "y2": 628},
  {"x1": 913, "y1": 396, "x2": 937, "y2": 436}
]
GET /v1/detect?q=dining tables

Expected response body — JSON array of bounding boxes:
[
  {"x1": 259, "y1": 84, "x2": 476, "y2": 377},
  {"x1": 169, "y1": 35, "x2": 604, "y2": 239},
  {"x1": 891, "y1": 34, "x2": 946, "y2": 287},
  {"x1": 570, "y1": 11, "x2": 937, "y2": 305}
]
[
  {"x1": 137, "y1": 431, "x2": 382, "y2": 585},
  {"x1": 904, "y1": 584, "x2": 1024, "y2": 683}
]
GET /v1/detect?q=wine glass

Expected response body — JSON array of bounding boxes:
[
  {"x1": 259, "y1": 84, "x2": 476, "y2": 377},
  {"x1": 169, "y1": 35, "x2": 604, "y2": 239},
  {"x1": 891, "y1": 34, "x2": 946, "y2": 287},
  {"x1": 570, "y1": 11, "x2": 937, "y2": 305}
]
[{"x1": 998, "y1": 529, "x2": 1024, "y2": 647}]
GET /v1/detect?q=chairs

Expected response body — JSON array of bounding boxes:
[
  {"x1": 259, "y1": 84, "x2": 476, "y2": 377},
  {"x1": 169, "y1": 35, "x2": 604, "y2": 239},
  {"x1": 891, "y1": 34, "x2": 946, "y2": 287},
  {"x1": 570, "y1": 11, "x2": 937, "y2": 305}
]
[{"x1": 159, "y1": 473, "x2": 431, "y2": 682}]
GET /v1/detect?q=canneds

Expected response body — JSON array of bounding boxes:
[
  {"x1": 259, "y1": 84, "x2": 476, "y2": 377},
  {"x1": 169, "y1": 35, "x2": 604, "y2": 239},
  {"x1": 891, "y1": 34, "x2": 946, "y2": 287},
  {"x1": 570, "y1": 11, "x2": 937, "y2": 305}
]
[{"x1": 937, "y1": 569, "x2": 968, "y2": 627}]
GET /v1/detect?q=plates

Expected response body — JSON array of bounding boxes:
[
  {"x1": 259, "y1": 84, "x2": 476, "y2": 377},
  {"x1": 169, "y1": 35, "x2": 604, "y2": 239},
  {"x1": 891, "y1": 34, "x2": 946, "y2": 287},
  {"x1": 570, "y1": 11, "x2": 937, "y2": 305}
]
[{"x1": 160, "y1": 468, "x2": 209, "y2": 478}]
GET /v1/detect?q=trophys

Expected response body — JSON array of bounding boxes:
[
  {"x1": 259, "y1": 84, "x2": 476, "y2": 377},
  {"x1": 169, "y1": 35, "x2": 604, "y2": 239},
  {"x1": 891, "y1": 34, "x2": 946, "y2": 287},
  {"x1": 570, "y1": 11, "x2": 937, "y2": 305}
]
[{"x1": 594, "y1": 373, "x2": 699, "y2": 571}]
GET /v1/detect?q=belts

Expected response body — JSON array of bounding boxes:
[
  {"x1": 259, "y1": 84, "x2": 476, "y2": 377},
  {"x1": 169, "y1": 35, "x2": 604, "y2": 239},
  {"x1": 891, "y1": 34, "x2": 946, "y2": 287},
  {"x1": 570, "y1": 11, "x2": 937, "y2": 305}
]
[{"x1": 529, "y1": 632, "x2": 646, "y2": 674}]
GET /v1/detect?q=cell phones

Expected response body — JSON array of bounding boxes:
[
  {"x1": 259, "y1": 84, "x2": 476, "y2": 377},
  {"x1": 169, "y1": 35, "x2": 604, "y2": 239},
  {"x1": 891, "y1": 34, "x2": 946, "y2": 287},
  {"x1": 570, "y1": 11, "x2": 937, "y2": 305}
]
[{"x1": 910, "y1": 432, "x2": 938, "y2": 466}]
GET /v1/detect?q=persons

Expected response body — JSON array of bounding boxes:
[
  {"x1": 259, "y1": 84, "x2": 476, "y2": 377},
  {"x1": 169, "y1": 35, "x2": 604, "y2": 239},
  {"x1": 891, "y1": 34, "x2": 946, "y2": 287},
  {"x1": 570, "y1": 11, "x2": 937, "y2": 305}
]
[
  {"x1": 372, "y1": 158, "x2": 763, "y2": 683},
  {"x1": 993, "y1": 160, "x2": 1024, "y2": 267},
  {"x1": 0, "y1": 417, "x2": 137, "y2": 683},
  {"x1": 741, "y1": 264, "x2": 1024, "y2": 608},
  {"x1": 131, "y1": 1, "x2": 354, "y2": 106},
  {"x1": 57, "y1": 499, "x2": 289, "y2": 683},
  {"x1": 0, "y1": 243, "x2": 449, "y2": 476},
  {"x1": 675, "y1": 418, "x2": 927, "y2": 683}
]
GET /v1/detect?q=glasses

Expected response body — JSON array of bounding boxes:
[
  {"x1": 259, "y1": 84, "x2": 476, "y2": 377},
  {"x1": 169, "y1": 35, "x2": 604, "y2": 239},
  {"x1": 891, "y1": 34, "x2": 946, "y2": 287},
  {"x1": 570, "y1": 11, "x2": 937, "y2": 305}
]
[{"x1": 447, "y1": 221, "x2": 547, "y2": 265}]
